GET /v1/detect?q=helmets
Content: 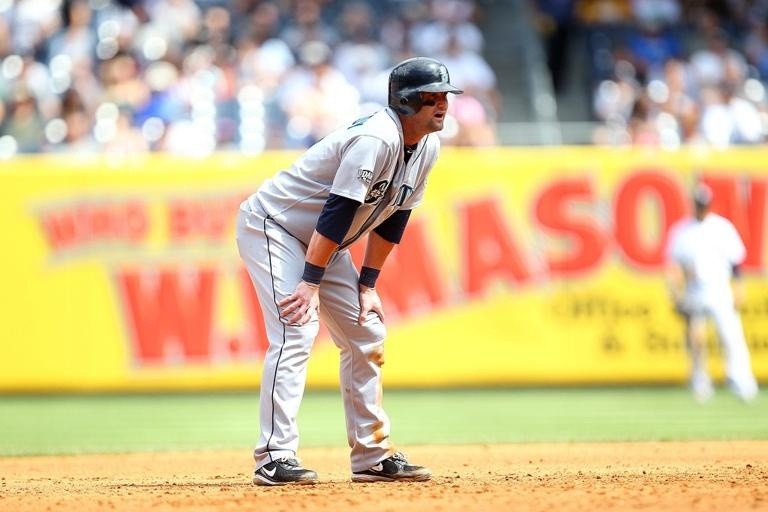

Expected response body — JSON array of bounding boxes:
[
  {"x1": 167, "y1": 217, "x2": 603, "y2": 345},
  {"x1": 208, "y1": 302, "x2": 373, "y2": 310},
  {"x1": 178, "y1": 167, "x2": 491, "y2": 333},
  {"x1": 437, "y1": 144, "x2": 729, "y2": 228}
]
[{"x1": 388, "y1": 56, "x2": 464, "y2": 117}]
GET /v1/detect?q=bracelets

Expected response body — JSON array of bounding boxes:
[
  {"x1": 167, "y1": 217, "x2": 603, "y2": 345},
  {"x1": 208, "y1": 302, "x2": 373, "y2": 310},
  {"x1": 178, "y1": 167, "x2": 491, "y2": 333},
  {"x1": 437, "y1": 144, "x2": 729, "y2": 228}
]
[
  {"x1": 302, "y1": 260, "x2": 326, "y2": 285},
  {"x1": 236, "y1": 56, "x2": 464, "y2": 485},
  {"x1": 358, "y1": 265, "x2": 381, "y2": 289}
]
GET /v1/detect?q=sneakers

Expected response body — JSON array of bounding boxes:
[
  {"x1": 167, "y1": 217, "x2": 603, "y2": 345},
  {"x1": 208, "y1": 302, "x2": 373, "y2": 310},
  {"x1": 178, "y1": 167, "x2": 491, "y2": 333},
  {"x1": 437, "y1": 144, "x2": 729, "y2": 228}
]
[
  {"x1": 253, "y1": 457, "x2": 318, "y2": 486},
  {"x1": 351, "y1": 451, "x2": 432, "y2": 482}
]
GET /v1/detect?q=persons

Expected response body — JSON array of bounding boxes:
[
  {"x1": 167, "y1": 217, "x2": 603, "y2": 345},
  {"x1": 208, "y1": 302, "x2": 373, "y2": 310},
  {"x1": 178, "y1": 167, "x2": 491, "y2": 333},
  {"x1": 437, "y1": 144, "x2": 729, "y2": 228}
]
[{"x1": 662, "y1": 183, "x2": 761, "y2": 406}]
